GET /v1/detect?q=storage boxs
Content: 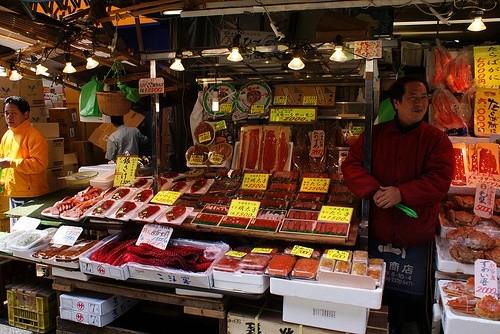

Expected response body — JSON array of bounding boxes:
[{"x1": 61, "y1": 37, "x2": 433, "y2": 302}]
[
  {"x1": 436, "y1": 214, "x2": 500, "y2": 334},
  {"x1": 7, "y1": 234, "x2": 389, "y2": 334},
  {"x1": 274, "y1": 84, "x2": 337, "y2": 107},
  {"x1": 0, "y1": 76, "x2": 92, "y2": 234},
  {"x1": 219, "y1": 30, "x2": 283, "y2": 70}
]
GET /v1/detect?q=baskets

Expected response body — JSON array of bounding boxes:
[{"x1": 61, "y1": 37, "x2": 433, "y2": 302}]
[{"x1": 97, "y1": 91, "x2": 132, "y2": 116}]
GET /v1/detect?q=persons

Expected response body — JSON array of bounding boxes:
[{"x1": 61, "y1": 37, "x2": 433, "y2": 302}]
[
  {"x1": 340, "y1": 74, "x2": 455, "y2": 334},
  {"x1": 105, "y1": 115, "x2": 150, "y2": 164},
  {"x1": 0, "y1": 95, "x2": 50, "y2": 234}
]
